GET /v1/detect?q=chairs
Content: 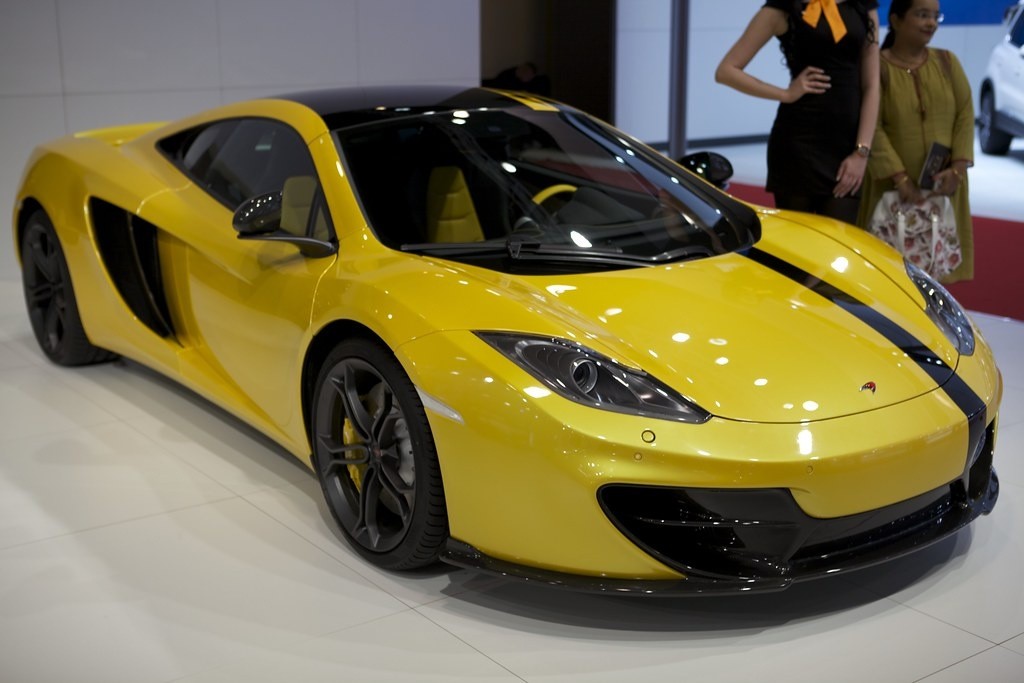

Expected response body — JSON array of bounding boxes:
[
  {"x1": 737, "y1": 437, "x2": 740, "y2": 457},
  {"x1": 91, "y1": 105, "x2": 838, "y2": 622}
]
[
  {"x1": 279, "y1": 177, "x2": 335, "y2": 243},
  {"x1": 426, "y1": 167, "x2": 484, "y2": 245}
]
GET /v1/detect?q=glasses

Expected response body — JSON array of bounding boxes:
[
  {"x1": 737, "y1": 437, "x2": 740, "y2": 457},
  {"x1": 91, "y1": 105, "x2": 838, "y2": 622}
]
[{"x1": 907, "y1": 10, "x2": 943, "y2": 23}]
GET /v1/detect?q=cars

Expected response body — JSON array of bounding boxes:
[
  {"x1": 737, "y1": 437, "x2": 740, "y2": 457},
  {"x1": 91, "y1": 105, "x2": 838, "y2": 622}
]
[{"x1": 972, "y1": 0, "x2": 1024, "y2": 156}]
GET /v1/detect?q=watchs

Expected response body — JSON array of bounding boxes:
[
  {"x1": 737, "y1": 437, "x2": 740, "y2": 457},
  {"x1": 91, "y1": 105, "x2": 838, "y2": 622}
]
[{"x1": 856, "y1": 144, "x2": 870, "y2": 157}]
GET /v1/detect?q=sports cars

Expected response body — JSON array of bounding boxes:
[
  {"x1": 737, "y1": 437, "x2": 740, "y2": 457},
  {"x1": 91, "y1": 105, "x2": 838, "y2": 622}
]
[{"x1": 8, "y1": 86, "x2": 1004, "y2": 601}]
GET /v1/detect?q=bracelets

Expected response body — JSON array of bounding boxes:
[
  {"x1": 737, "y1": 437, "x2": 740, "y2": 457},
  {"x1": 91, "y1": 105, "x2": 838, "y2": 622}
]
[
  {"x1": 953, "y1": 167, "x2": 963, "y2": 181},
  {"x1": 895, "y1": 175, "x2": 909, "y2": 189}
]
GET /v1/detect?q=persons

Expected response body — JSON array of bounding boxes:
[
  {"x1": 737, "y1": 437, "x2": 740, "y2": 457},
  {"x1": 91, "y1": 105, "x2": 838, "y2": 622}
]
[
  {"x1": 859, "y1": 0, "x2": 974, "y2": 284},
  {"x1": 715, "y1": 0, "x2": 878, "y2": 225}
]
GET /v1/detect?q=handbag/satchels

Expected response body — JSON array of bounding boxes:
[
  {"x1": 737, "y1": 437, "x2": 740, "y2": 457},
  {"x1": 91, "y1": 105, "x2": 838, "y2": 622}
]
[{"x1": 868, "y1": 187, "x2": 963, "y2": 282}]
